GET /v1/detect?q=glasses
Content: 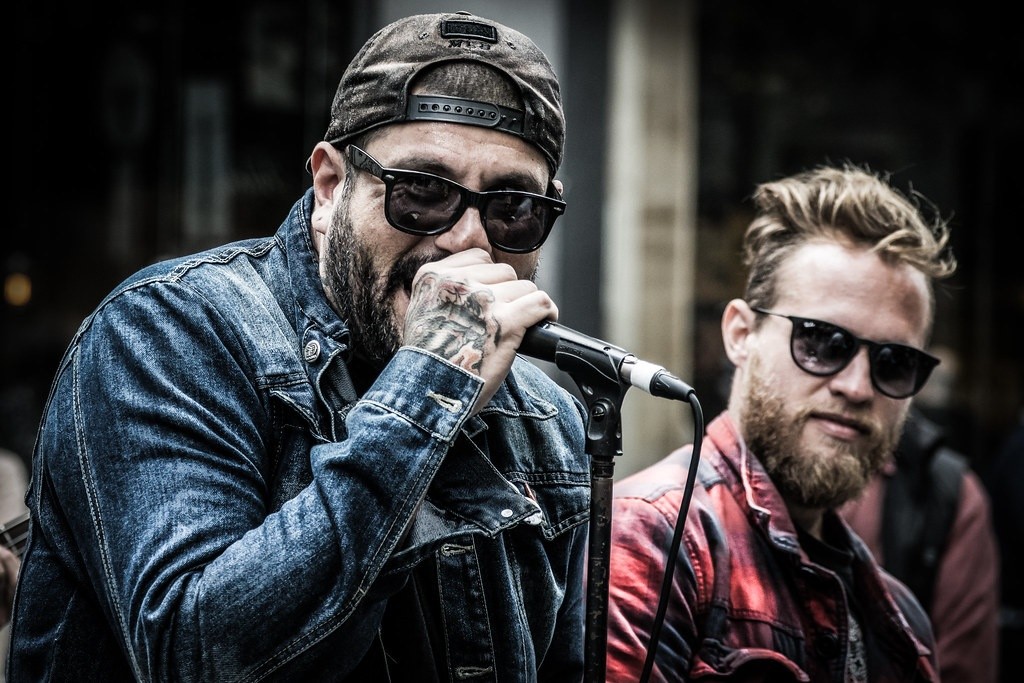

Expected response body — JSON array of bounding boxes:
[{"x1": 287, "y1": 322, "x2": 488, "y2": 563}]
[
  {"x1": 750, "y1": 307, "x2": 942, "y2": 399},
  {"x1": 339, "y1": 143, "x2": 566, "y2": 255}
]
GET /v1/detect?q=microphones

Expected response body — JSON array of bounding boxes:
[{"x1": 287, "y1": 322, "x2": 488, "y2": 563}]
[{"x1": 515, "y1": 318, "x2": 695, "y2": 403}]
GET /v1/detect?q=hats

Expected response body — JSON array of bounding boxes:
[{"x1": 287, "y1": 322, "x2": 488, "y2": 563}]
[{"x1": 305, "y1": 9, "x2": 568, "y2": 176}]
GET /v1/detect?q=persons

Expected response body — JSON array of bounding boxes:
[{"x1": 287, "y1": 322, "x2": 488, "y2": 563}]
[
  {"x1": 6, "y1": 10, "x2": 594, "y2": 683},
  {"x1": 605, "y1": 161, "x2": 999, "y2": 683}
]
